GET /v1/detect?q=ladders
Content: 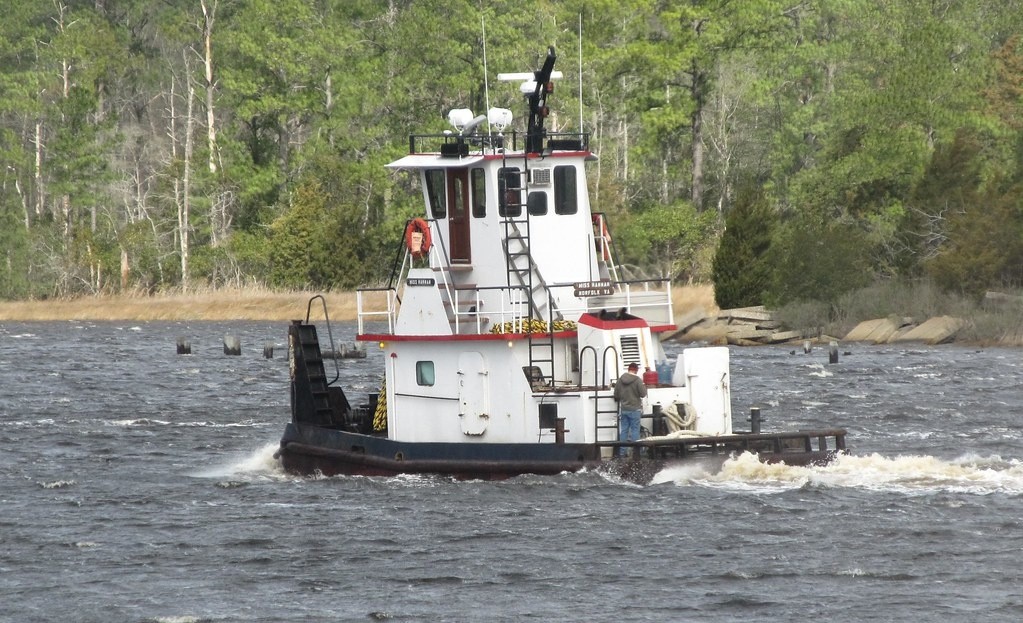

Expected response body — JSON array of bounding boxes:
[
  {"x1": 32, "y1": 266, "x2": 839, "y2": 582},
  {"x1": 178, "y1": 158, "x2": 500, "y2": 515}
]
[{"x1": 501, "y1": 217, "x2": 565, "y2": 324}]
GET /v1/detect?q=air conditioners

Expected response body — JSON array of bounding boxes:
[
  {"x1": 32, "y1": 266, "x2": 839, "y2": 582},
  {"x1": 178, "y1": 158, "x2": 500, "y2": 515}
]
[{"x1": 528, "y1": 167, "x2": 551, "y2": 186}]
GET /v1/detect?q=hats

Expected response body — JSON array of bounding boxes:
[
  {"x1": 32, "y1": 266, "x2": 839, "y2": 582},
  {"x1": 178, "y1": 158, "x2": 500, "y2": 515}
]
[{"x1": 628, "y1": 363, "x2": 638, "y2": 372}]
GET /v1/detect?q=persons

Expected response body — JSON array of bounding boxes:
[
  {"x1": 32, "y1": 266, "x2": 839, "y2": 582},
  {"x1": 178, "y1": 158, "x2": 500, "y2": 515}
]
[{"x1": 613, "y1": 363, "x2": 648, "y2": 457}]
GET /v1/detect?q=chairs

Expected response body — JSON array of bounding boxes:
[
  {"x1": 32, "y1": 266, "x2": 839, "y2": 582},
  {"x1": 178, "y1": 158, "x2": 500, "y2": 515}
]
[{"x1": 522, "y1": 366, "x2": 552, "y2": 392}]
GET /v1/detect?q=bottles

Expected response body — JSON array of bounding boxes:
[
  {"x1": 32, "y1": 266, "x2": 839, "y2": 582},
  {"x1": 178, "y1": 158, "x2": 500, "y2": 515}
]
[{"x1": 655, "y1": 359, "x2": 676, "y2": 385}]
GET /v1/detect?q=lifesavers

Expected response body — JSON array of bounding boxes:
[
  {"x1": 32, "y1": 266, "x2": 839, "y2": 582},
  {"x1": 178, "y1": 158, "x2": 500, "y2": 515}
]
[
  {"x1": 405, "y1": 217, "x2": 432, "y2": 259},
  {"x1": 592, "y1": 213, "x2": 608, "y2": 261}
]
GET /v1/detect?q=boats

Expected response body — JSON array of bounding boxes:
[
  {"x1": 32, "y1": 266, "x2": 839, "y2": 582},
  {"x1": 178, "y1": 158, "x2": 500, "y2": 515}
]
[{"x1": 272, "y1": 10, "x2": 850, "y2": 481}]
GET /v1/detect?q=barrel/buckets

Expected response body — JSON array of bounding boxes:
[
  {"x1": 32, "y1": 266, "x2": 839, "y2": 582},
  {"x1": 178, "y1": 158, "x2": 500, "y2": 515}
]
[{"x1": 643, "y1": 371, "x2": 657, "y2": 386}]
